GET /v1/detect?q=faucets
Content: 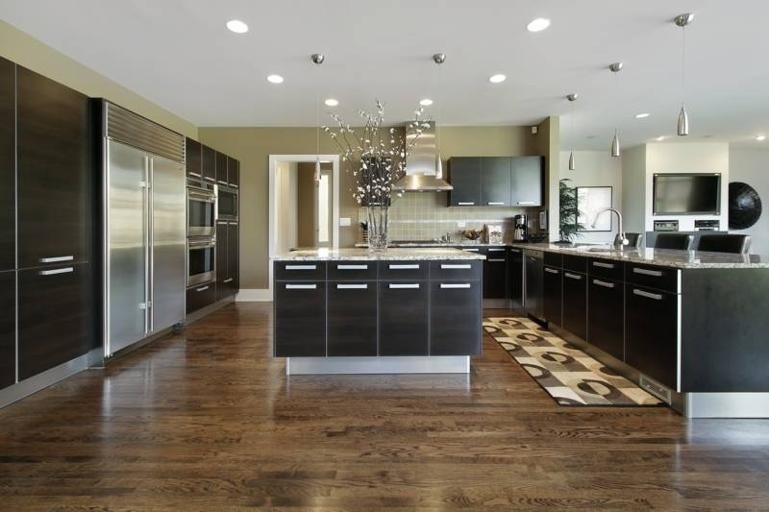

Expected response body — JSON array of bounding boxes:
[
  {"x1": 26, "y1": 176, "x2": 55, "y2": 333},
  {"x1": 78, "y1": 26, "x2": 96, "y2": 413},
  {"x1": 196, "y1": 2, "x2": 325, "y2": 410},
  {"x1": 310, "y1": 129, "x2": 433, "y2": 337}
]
[{"x1": 591, "y1": 209, "x2": 629, "y2": 250}]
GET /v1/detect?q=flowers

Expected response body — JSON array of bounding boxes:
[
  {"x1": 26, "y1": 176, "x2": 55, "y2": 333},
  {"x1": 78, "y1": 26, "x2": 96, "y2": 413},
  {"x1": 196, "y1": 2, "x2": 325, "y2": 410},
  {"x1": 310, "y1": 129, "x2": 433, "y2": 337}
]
[{"x1": 322, "y1": 102, "x2": 430, "y2": 246}]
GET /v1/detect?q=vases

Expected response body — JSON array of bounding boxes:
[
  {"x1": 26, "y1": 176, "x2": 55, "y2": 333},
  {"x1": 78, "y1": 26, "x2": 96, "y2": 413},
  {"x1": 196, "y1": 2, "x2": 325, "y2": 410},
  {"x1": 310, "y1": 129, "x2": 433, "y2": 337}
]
[{"x1": 366, "y1": 200, "x2": 390, "y2": 254}]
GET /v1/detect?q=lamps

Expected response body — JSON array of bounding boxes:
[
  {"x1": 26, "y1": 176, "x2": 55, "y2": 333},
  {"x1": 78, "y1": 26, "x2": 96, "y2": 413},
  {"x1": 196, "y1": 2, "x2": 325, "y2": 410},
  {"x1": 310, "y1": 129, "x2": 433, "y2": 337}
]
[
  {"x1": 609, "y1": 63, "x2": 624, "y2": 158},
  {"x1": 568, "y1": 93, "x2": 579, "y2": 170},
  {"x1": 434, "y1": 53, "x2": 446, "y2": 179},
  {"x1": 675, "y1": 13, "x2": 696, "y2": 135},
  {"x1": 311, "y1": 54, "x2": 325, "y2": 182}
]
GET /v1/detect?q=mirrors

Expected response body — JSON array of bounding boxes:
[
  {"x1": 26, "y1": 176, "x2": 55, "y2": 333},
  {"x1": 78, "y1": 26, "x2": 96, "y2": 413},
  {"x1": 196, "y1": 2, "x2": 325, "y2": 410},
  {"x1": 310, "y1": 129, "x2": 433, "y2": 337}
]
[{"x1": 575, "y1": 185, "x2": 612, "y2": 232}]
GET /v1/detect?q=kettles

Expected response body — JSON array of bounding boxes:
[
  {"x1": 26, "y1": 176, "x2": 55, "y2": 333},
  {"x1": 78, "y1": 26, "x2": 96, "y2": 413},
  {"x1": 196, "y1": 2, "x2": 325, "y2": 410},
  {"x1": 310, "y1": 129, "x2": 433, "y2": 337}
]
[{"x1": 512, "y1": 214, "x2": 528, "y2": 240}]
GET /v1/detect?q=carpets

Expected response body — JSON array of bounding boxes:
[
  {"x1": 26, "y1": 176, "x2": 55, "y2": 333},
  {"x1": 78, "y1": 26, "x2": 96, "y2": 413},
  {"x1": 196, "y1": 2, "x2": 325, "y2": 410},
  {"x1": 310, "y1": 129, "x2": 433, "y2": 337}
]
[{"x1": 483, "y1": 314, "x2": 665, "y2": 406}]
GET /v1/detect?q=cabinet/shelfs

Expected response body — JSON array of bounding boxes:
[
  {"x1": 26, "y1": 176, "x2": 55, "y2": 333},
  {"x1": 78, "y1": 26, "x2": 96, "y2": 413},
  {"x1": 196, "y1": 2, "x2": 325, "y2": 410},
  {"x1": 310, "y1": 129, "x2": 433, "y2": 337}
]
[
  {"x1": 543, "y1": 252, "x2": 681, "y2": 390},
  {"x1": 481, "y1": 156, "x2": 510, "y2": 207},
  {"x1": 462, "y1": 247, "x2": 524, "y2": 303},
  {"x1": 186, "y1": 135, "x2": 240, "y2": 187},
  {"x1": 511, "y1": 156, "x2": 544, "y2": 208},
  {"x1": 186, "y1": 281, "x2": 217, "y2": 315},
  {"x1": 0, "y1": 57, "x2": 106, "y2": 406},
  {"x1": 273, "y1": 261, "x2": 483, "y2": 356},
  {"x1": 447, "y1": 157, "x2": 481, "y2": 206},
  {"x1": 217, "y1": 221, "x2": 239, "y2": 299}
]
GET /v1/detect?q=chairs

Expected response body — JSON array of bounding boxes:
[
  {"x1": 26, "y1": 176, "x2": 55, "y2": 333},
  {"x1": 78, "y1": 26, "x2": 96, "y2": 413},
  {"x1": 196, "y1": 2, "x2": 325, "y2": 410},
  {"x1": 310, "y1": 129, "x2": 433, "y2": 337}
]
[{"x1": 613, "y1": 234, "x2": 752, "y2": 255}]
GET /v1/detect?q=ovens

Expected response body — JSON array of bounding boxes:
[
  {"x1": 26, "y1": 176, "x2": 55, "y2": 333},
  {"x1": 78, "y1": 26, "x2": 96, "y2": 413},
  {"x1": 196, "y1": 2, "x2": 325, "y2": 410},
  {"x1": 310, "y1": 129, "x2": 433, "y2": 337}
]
[{"x1": 185, "y1": 176, "x2": 217, "y2": 290}]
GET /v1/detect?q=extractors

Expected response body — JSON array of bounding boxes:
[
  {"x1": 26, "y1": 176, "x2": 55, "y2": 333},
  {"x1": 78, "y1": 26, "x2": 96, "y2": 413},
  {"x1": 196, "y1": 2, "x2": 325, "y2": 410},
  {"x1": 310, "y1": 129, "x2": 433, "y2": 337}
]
[{"x1": 388, "y1": 120, "x2": 454, "y2": 192}]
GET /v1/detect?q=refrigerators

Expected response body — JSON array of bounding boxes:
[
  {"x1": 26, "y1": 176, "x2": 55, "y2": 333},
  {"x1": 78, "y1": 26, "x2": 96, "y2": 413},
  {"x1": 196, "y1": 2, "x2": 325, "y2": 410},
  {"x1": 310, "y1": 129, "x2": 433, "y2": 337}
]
[{"x1": 98, "y1": 96, "x2": 189, "y2": 357}]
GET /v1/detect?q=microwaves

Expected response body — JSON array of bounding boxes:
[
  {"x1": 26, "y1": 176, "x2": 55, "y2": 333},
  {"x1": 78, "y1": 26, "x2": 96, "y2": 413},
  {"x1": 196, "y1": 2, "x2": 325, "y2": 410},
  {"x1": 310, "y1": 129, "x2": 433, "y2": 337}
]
[{"x1": 214, "y1": 184, "x2": 239, "y2": 224}]
